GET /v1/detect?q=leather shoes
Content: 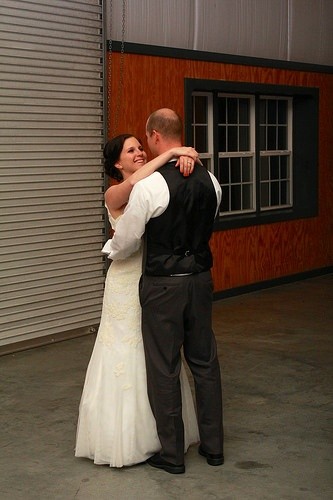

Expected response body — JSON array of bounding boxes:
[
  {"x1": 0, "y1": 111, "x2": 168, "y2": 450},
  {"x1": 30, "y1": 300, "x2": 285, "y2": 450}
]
[
  {"x1": 146, "y1": 452, "x2": 185, "y2": 473},
  {"x1": 198, "y1": 443, "x2": 224, "y2": 466}
]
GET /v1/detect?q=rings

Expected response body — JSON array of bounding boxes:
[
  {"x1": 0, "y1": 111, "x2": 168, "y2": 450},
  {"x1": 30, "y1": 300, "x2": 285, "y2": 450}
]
[{"x1": 187, "y1": 161, "x2": 191, "y2": 165}]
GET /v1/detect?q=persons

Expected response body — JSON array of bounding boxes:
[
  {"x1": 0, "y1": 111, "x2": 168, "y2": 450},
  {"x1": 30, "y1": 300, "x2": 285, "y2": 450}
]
[
  {"x1": 95, "y1": 133, "x2": 199, "y2": 464},
  {"x1": 99, "y1": 106, "x2": 225, "y2": 474}
]
